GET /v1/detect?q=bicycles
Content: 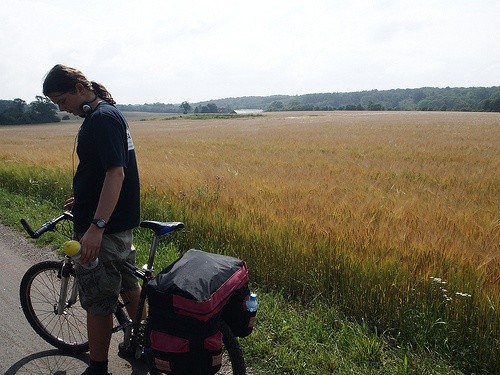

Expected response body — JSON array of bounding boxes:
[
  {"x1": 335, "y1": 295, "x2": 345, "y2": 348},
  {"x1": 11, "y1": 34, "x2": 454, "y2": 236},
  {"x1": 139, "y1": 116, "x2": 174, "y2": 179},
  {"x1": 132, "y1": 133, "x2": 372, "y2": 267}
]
[{"x1": 21, "y1": 208, "x2": 246, "y2": 375}]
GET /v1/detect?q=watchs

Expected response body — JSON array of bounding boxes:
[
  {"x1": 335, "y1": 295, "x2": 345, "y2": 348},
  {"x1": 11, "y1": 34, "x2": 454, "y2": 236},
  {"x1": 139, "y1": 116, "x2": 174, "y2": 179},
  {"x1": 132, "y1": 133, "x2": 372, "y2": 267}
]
[{"x1": 92, "y1": 218, "x2": 109, "y2": 229}]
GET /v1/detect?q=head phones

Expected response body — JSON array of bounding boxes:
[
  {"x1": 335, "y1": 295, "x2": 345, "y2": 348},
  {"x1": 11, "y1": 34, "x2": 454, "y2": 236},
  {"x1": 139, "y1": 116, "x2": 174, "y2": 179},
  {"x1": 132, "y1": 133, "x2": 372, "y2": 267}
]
[{"x1": 79, "y1": 96, "x2": 102, "y2": 118}]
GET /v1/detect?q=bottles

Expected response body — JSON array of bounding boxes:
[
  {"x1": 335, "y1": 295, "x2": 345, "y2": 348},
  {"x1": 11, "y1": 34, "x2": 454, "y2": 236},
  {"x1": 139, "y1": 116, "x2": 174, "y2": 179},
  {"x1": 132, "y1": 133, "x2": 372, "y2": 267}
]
[
  {"x1": 246, "y1": 294, "x2": 257, "y2": 327},
  {"x1": 63, "y1": 240, "x2": 98, "y2": 269}
]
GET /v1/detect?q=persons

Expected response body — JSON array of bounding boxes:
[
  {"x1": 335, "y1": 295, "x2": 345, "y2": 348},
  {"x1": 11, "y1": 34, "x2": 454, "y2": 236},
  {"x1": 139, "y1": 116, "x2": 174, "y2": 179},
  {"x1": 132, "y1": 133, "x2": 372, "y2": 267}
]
[{"x1": 42, "y1": 64, "x2": 141, "y2": 375}]
